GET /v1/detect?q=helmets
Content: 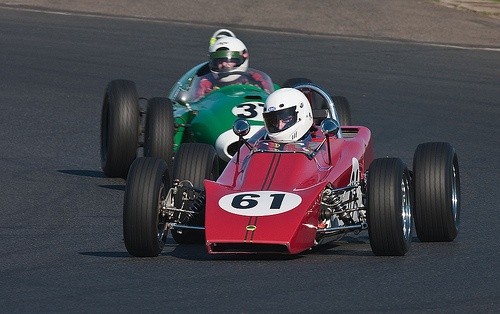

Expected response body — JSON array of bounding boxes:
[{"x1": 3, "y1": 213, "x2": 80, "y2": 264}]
[
  {"x1": 262, "y1": 87, "x2": 313, "y2": 143},
  {"x1": 209, "y1": 29, "x2": 235, "y2": 45},
  {"x1": 207, "y1": 37, "x2": 249, "y2": 83}
]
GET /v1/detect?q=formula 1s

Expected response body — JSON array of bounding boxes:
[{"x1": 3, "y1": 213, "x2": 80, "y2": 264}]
[{"x1": 100, "y1": 60, "x2": 462, "y2": 257}]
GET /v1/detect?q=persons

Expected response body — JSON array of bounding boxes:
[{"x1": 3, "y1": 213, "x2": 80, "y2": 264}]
[
  {"x1": 197, "y1": 36, "x2": 272, "y2": 102},
  {"x1": 257, "y1": 87, "x2": 319, "y2": 153}
]
